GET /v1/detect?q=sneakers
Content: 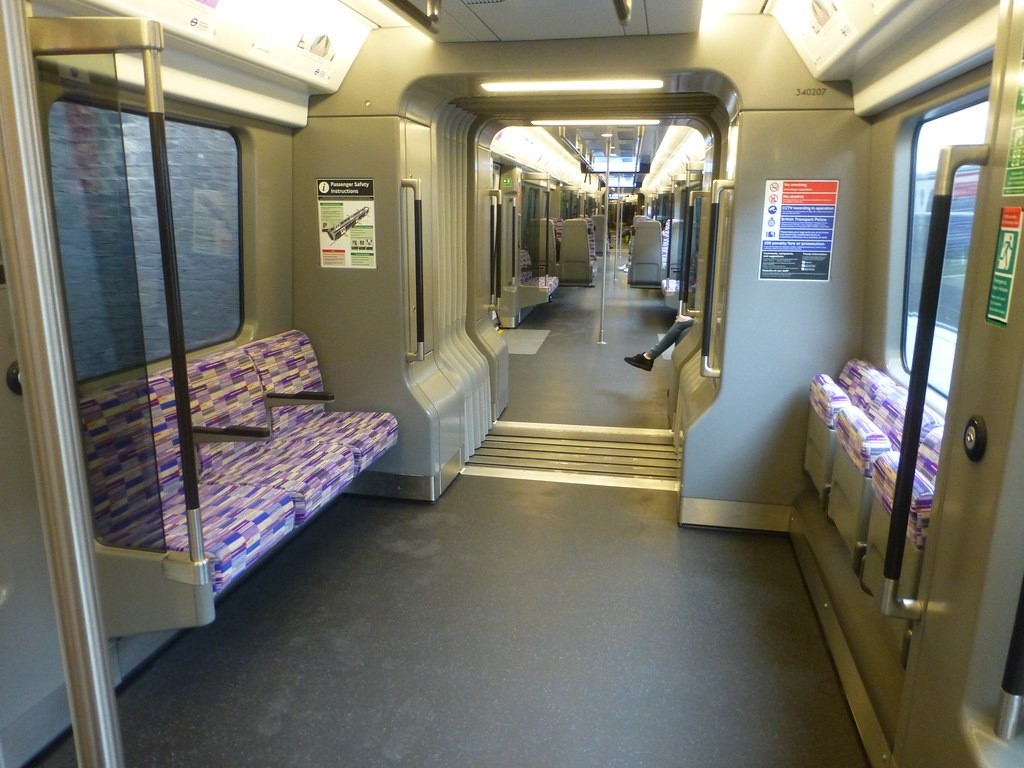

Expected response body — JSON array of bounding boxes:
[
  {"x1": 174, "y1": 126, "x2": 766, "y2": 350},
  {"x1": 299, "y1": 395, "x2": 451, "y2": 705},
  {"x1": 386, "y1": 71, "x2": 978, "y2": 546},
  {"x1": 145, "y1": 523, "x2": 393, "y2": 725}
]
[{"x1": 624, "y1": 352, "x2": 654, "y2": 372}]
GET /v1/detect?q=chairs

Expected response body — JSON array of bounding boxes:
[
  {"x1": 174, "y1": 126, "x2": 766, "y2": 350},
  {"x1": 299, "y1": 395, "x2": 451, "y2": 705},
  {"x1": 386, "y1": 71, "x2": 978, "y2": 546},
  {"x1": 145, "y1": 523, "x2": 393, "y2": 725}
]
[
  {"x1": 79, "y1": 329, "x2": 398, "y2": 599},
  {"x1": 519, "y1": 249, "x2": 559, "y2": 295},
  {"x1": 804, "y1": 356, "x2": 946, "y2": 669},
  {"x1": 628, "y1": 216, "x2": 684, "y2": 296},
  {"x1": 527, "y1": 216, "x2": 609, "y2": 287}
]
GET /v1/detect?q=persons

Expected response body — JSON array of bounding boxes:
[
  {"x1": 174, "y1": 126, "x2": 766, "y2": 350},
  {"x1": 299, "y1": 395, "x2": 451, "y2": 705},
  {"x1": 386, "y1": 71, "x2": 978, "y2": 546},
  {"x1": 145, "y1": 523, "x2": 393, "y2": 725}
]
[
  {"x1": 623, "y1": 315, "x2": 693, "y2": 371},
  {"x1": 618, "y1": 240, "x2": 632, "y2": 273}
]
